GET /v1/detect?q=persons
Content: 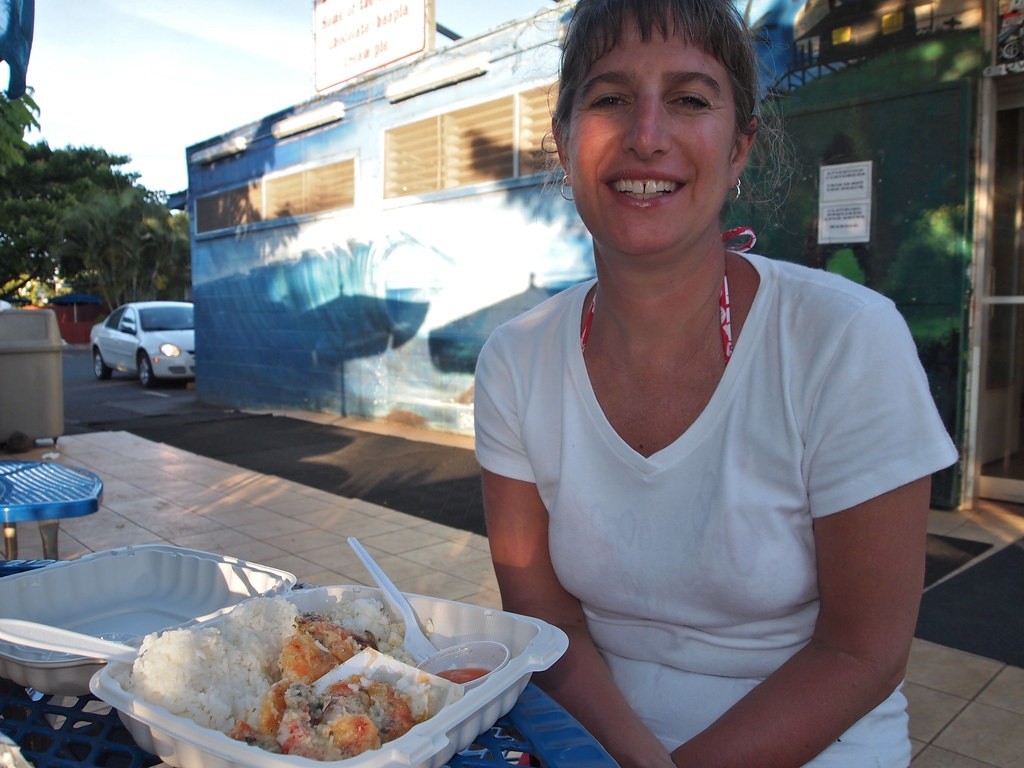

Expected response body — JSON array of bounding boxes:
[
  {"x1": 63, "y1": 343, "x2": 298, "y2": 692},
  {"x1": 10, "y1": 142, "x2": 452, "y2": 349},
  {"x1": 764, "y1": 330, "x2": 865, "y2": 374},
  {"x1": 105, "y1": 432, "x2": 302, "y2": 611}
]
[{"x1": 473, "y1": 1, "x2": 960, "y2": 768}]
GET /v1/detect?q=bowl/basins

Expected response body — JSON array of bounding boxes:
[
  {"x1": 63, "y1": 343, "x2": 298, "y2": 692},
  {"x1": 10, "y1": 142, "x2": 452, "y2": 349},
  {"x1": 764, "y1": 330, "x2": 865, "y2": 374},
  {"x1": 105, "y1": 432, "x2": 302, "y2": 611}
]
[
  {"x1": 416, "y1": 639, "x2": 511, "y2": 692},
  {"x1": 90, "y1": 584, "x2": 570, "y2": 768},
  {"x1": 0, "y1": 543, "x2": 296, "y2": 696}
]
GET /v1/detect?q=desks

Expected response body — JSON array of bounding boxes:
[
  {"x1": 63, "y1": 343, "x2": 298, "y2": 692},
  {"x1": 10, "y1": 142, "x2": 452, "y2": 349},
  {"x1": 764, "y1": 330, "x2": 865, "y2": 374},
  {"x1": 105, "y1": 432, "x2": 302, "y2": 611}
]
[
  {"x1": 0, "y1": 557, "x2": 621, "y2": 768},
  {"x1": 0, "y1": 456, "x2": 103, "y2": 561}
]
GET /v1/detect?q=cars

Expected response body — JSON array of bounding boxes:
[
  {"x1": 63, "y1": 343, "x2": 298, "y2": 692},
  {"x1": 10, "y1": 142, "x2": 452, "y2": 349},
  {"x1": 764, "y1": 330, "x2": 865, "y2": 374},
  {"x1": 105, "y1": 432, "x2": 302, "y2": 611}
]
[{"x1": 89, "y1": 302, "x2": 195, "y2": 388}]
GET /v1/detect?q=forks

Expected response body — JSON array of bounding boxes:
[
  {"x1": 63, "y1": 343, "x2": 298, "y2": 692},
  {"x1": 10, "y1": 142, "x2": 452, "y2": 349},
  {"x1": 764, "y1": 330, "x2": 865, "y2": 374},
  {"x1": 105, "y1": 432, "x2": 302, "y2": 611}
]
[{"x1": 346, "y1": 536, "x2": 439, "y2": 664}]
